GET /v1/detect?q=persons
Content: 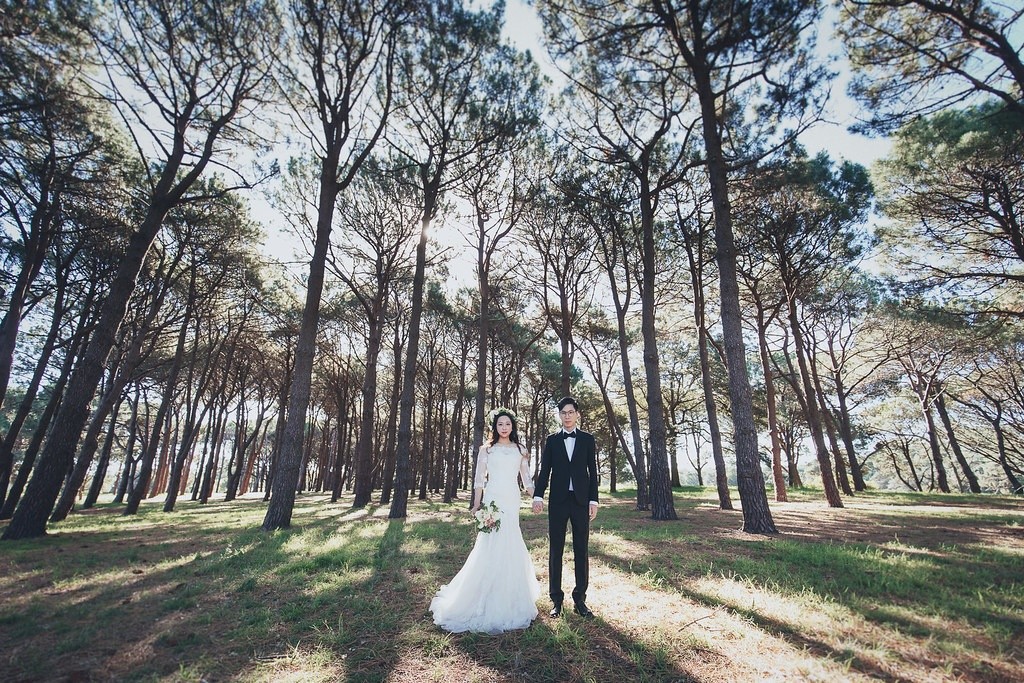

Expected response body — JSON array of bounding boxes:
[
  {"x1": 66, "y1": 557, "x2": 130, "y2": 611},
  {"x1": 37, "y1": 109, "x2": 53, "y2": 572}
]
[
  {"x1": 429, "y1": 406, "x2": 547, "y2": 634},
  {"x1": 532, "y1": 397, "x2": 599, "y2": 617}
]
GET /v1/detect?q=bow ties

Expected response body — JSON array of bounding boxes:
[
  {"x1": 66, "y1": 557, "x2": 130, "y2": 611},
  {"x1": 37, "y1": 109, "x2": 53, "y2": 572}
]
[{"x1": 563, "y1": 431, "x2": 576, "y2": 439}]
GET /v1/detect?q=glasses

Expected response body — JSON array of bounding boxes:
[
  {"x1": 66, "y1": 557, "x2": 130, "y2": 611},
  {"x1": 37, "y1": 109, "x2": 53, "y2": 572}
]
[{"x1": 560, "y1": 410, "x2": 577, "y2": 418}]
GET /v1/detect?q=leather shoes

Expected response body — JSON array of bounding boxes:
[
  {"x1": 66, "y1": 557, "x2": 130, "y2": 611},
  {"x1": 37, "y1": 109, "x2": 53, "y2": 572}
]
[
  {"x1": 573, "y1": 601, "x2": 594, "y2": 619},
  {"x1": 550, "y1": 602, "x2": 564, "y2": 617}
]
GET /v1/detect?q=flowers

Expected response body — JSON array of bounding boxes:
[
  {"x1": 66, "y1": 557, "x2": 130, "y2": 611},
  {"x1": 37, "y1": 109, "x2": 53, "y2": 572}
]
[
  {"x1": 487, "y1": 406, "x2": 519, "y2": 424},
  {"x1": 473, "y1": 501, "x2": 505, "y2": 533}
]
